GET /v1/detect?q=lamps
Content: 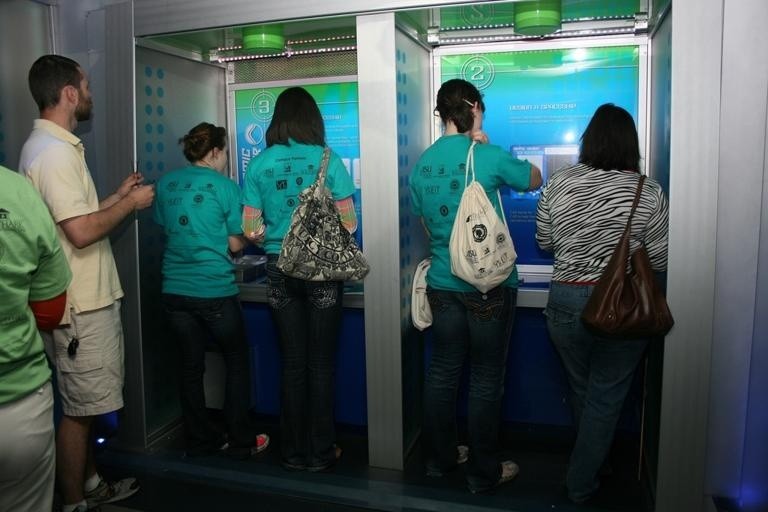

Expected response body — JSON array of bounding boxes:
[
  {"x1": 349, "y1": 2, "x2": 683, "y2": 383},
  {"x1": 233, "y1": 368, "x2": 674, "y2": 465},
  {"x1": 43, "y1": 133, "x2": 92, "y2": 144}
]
[
  {"x1": 242, "y1": 23, "x2": 285, "y2": 54},
  {"x1": 514, "y1": 0, "x2": 562, "y2": 36}
]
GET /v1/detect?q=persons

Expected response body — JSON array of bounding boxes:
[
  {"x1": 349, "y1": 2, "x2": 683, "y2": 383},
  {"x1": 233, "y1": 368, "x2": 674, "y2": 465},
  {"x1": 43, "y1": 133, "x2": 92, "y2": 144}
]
[
  {"x1": 149, "y1": 120, "x2": 270, "y2": 461},
  {"x1": 536, "y1": 102, "x2": 669, "y2": 511},
  {"x1": 16, "y1": 53, "x2": 159, "y2": 512},
  {"x1": 240, "y1": 87, "x2": 359, "y2": 473},
  {"x1": 407, "y1": 78, "x2": 545, "y2": 494},
  {"x1": 0, "y1": 165, "x2": 75, "y2": 511}
]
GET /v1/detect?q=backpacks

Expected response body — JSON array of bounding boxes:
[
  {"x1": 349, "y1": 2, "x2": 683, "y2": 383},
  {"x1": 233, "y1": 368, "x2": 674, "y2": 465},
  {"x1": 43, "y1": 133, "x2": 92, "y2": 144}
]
[{"x1": 448, "y1": 181, "x2": 518, "y2": 294}]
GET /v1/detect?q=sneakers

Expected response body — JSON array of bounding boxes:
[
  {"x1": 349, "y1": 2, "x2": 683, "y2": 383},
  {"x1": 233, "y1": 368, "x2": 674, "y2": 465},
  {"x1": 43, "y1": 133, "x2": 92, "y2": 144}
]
[
  {"x1": 249, "y1": 433, "x2": 269, "y2": 457},
  {"x1": 306, "y1": 442, "x2": 344, "y2": 474},
  {"x1": 469, "y1": 460, "x2": 520, "y2": 494},
  {"x1": 428, "y1": 446, "x2": 469, "y2": 477},
  {"x1": 85, "y1": 477, "x2": 140, "y2": 507}
]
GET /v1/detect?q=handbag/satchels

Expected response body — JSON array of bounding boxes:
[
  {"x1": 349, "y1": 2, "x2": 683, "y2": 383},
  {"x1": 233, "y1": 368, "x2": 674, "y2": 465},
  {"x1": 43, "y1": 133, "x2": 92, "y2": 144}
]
[
  {"x1": 581, "y1": 220, "x2": 674, "y2": 337},
  {"x1": 277, "y1": 186, "x2": 371, "y2": 282}
]
